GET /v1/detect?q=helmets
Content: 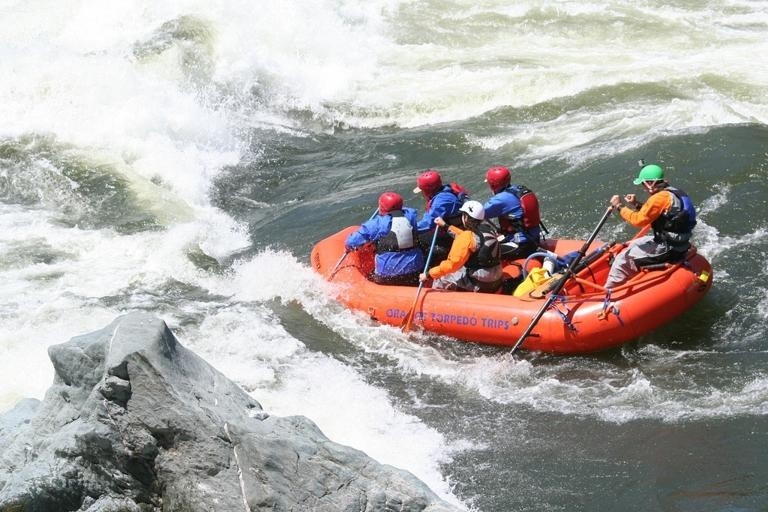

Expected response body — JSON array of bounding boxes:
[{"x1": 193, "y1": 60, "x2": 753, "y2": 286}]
[
  {"x1": 415, "y1": 169, "x2": 441, "y2": 196},
  {"x1": 632, "y1": 164, "x2": 664, "y2": 187},
  {"x1": 377, "y1": 190, "x2": 403, "y2": 215},
  {"x1": 457, "y1": 200, "x2": 485, "y2": 221},
  {"x1": 484, "y1": 165, "x2": 512, "y2": 195}
]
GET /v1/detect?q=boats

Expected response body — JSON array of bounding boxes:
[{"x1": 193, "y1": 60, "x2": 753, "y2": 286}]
[{"x1": 309, "y1": 222, "x2": 714, "y2": 353}]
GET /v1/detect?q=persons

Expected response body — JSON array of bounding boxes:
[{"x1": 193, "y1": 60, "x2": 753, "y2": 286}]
[
  {"x1": 604, "y1": 164, "x2": 698, "y2": 287},
  {"x1": 483, "y1": 165, "x2": 540, "y2": 262},
  {"x1": 345, "y1": 192, "x2": 426, "y2": 285},
  {"x1": 413, "y1": 172, "x2": 472, "y2": 268},
  {"x1": 417, "y1": 200, "x2": 504, "y2": 293}
]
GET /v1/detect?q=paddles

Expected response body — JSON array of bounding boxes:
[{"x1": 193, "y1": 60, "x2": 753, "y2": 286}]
[{"x1": 400, "y1": 225, "x2": 439, "y2": 335}]
